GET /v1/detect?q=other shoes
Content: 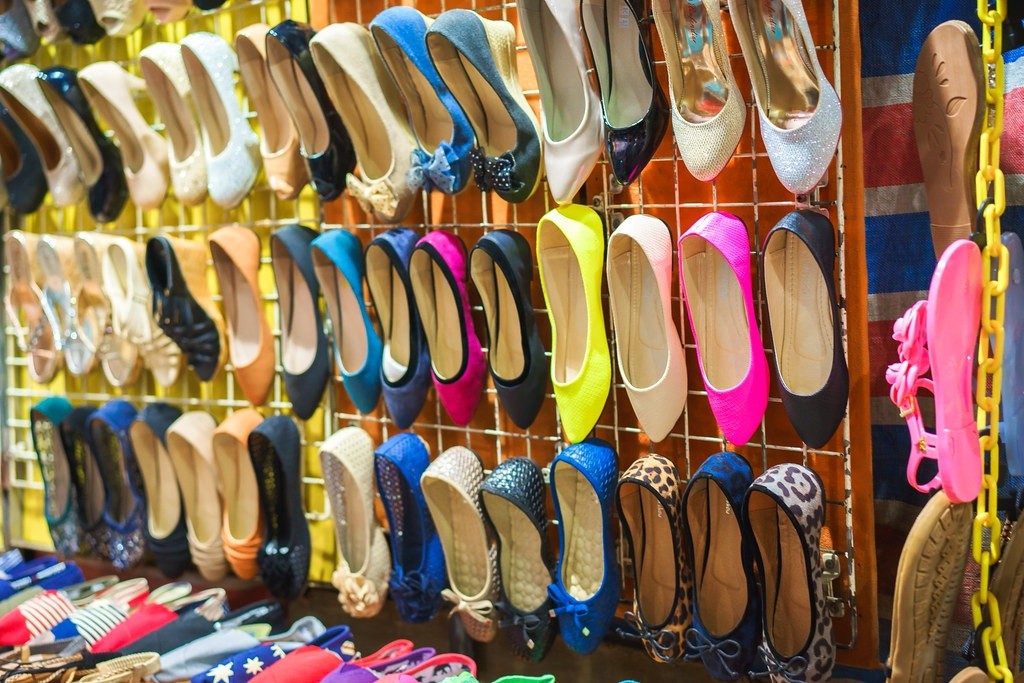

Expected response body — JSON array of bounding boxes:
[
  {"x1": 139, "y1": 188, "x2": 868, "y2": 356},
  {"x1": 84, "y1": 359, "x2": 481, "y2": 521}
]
[{"x1": 0, "y1": 396, "x2": 838, "y2": 683}]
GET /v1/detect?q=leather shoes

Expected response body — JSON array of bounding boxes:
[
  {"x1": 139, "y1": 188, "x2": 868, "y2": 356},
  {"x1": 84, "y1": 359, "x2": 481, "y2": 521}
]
[
  {"x1": 0, "y1": 0, "x2": 842, "y2": 223},
  {"x1": 0, "y1": 205, "x2": 849, "y2": 452}
]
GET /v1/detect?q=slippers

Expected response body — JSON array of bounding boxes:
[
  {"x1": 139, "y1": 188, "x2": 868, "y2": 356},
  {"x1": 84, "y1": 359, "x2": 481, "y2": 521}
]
[{"x1": 886, "y1": 21, "x2": 1024, "y2": 683}]
